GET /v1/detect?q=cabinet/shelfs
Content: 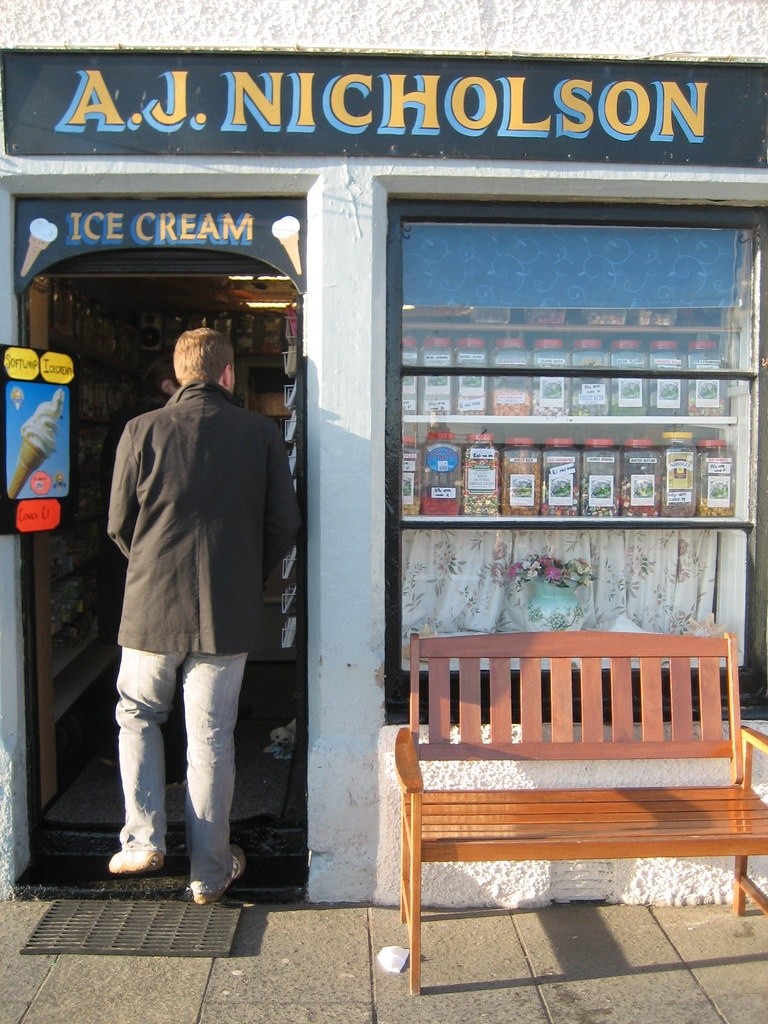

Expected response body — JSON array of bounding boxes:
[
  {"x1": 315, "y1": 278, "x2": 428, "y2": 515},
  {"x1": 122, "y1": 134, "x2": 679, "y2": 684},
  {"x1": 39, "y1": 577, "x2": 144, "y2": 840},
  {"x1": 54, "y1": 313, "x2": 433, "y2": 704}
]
[
  {"x1": 30, "y1": 277, "x2": 751, "y2": 810},
  {"x1": 400, "y1": 220, "x2": 759, "y2": 679}
]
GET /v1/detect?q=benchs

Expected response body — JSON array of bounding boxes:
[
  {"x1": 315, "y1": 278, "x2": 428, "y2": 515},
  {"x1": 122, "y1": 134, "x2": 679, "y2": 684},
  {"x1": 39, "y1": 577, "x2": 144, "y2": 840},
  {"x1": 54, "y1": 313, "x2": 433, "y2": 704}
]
[{"x1": 396, "y1": 633, "x2": 766, "y2": 996}]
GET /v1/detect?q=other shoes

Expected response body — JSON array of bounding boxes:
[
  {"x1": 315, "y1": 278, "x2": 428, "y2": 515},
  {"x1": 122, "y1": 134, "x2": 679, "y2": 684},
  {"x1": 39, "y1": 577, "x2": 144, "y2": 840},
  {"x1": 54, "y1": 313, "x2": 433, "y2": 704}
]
[
  {"x1": 108, "y1": 849, "x2": 163, "y2": 874},
  {"x1": 192, "y1": 844, "x2": 246, "y2": 905}
]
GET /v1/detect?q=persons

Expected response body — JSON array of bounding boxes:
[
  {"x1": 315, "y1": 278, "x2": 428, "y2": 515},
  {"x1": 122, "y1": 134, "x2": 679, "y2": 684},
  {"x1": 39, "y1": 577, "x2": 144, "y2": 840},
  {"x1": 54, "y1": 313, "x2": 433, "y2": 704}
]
[{"x1": 94, "y1": 326, "x2": 302, "y2": 906}]
